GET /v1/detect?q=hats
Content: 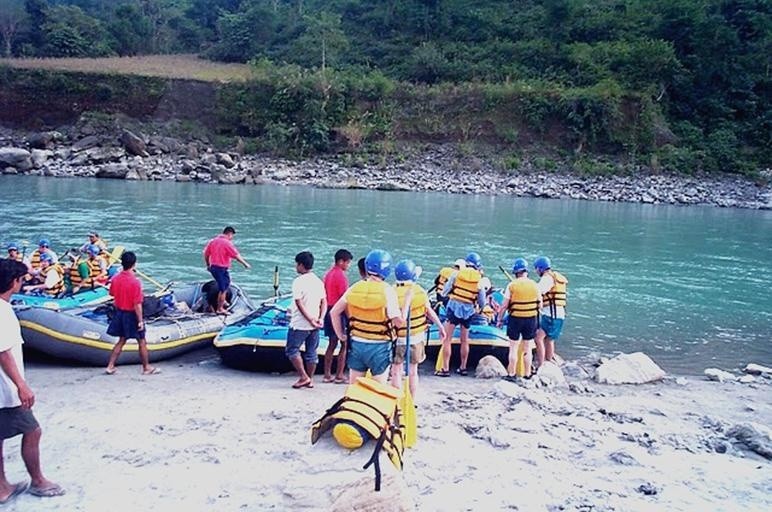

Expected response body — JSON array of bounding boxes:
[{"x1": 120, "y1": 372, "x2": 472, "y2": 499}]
[{"x1": 87, "y1": 230, "x2": 98, "y2": 236}]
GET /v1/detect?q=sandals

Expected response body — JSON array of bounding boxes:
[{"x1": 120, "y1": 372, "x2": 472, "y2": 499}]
[
  {"x1": 435, "y1": 369, "x2": 451, "y2": 377},
  {"x1": 454, "y1": 367, "x2": 469, "y2": 376}
]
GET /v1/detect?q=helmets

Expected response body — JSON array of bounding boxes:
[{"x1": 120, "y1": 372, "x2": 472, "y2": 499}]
[
  {"x1": 86, "y1": 244, "x2": 100, "y2": 256},
  {"x1": 454, "y1": 252, "x2": 482, "y2": 269},
  {"x1": 68, "y1": 248, "x2": 82, "y2": 259},
  {"x1": 40, "y1": 252, "x2": 52, "y2": 263},
  {"x1": 7, "y1": 242, "x2": 18, "y2": 252},
  {"x1": 394, "y1": 259, "x2": 422, "y2": 281},
  {"x1": 364, "y1": 249, "x2": 393, "y2": 279},
  {"x1": 533, "y1": 255, "x2": 552, "y2": 274},
  {"x1": 480, "y1": 276, "x2": 492, "y2": 294},
  {"x1": 39, "y1": 239, "x2": 49, "y2": 246},
  {"x1": 512, "y1": 257, "x2": 529, "y2": 274}
]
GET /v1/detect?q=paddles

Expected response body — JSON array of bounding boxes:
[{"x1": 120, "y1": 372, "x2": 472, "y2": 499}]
[
  {"x1": 402, "y1": 306, "x2": 417, "y2": 447},
  {"x1": 517, "y1": 340, "x2": 526, "y2": 376},
  {"x1": 107, "y1": 246, "x2": 125, "y2": 270},
  {"x1": 435, "y1": 344, "x2": 445, "y2": 371}
]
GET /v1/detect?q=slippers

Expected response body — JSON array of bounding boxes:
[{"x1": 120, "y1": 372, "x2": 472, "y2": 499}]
[
  {"x1": 217, "y1": 309, "x2": 231, "y2": 315},
  {"x1": 142, "y1": 367, "x2": 161, "y2": 375},
  {"x1": 335, "y1": 377, "x2": 349, "y2": 383},
  {"x1": 322, "y1": 375, "x2": 335, "y2": 383},
  {"x1": 29, "y1": 483, "x2": 66, "y2": 497},
  {"x1": 106, "y1": 367, "x2": 117, "y2": 374},
  {"x1": 1, "y1": 479, "x2": 27, "y2": 504},
  {"x1": 292, "y1": 378, "x2": 314, "y2": 388}
]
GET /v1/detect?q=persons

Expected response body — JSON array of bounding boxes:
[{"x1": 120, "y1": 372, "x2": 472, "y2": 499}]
[
  {"x1": 7, "y1": 231, "x2": 108, "y2": 296},
  {"x1": 497, "y1": 256, "x2": 568, "y2": 382},
  {"x1": 322, "y1": 249, "x2": 354, "y2": 383},
  {"x1": 331, "y1": 249, "x2": 447, "y2": 401},
  {"x1": 1, "y1": 256, "x2": 66, "y2": 506},
  {"x1": 286, "y1": 251, "x2": 328, "y2": 390},
  {"x1": 204, "y1": 227, "x2": 250, "y2": 315},
  {"x1": 434, "y1": 253, "x2": 493, "y2": 377},
  {"x1": 105, "y1": 251, "x2": 160, "y2": 375}
]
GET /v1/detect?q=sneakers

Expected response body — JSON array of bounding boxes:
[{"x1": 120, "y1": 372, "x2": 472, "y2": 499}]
[{"x1": 501, "y1": 375, "x2": 517, "y2": 382}]
